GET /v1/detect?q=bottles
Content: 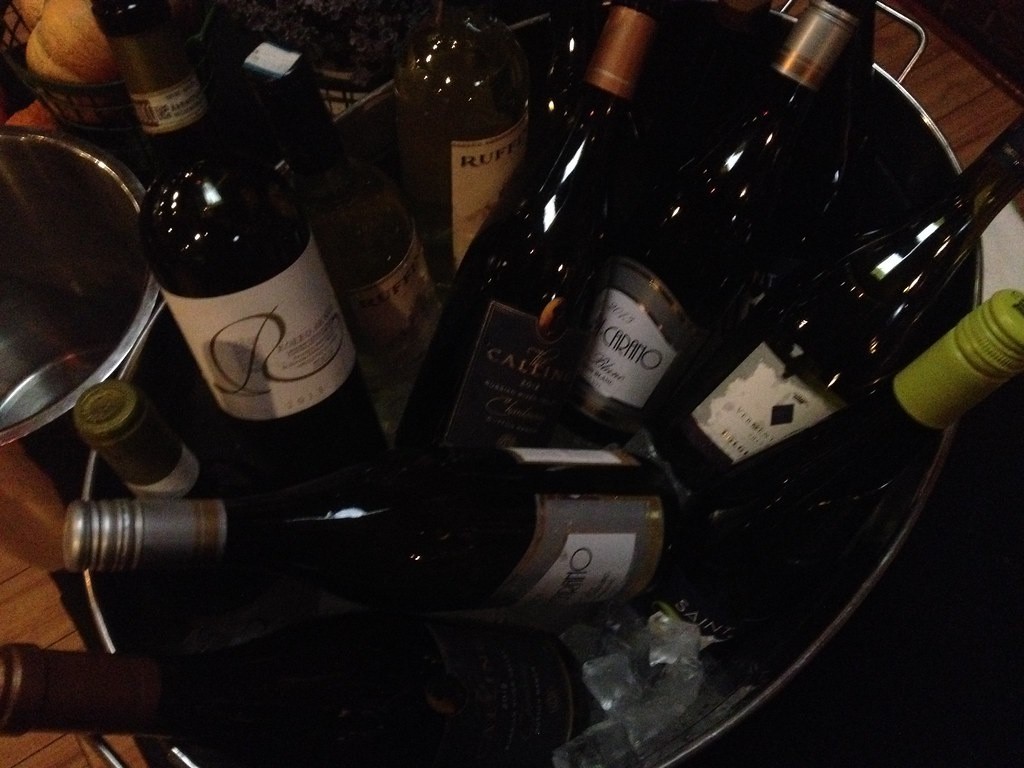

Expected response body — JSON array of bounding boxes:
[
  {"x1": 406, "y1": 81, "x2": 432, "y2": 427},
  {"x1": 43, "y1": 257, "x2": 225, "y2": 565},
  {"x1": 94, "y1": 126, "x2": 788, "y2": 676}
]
[
  {"x1": 62, "y1": 447, "x2": 684, "y2": 608},
  {"x1": 390, "y1": 0, "x2": 658, "y2": 558},
  {"x1": 275, "y1": 58, "x2": 442, "y2": 383},
  {"x1": 74, "y1": 382, "x2": 220, "y2": 502},
  {"x1": 669, "y1": 110, "x2": 1024, "y2": 486},
  {"x1": 92, "y1": 0, "x2": 393, "y2": 477},
  {"x1": 549, "y1": 0, "x2": 857, "y2": 441},
  {"x1": 0, "y1": 628, "x2": 586, "y2": 768},
  {"x1": 402, "y1": 0, "x2": 531, "y2": 297},
  {"x1": 641, "y1": 291, "x2": 1024, "y2": 660}
]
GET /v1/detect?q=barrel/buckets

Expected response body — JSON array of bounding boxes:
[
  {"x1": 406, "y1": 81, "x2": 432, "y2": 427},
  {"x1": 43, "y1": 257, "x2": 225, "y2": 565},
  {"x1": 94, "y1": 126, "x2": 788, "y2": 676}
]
[
  {"x1": 79, "y1": 0, "x2": 980, "y2": 768},
  {"x1": 0, "y1": 126, "x2": 162, "y2": 442}
]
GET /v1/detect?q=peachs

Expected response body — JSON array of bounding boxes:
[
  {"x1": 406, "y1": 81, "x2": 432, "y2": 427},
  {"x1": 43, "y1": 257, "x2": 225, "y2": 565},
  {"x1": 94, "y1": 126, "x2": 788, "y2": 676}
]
[{"x1": 27, "y1": 0, "x2": 114, "y2": 83}]
